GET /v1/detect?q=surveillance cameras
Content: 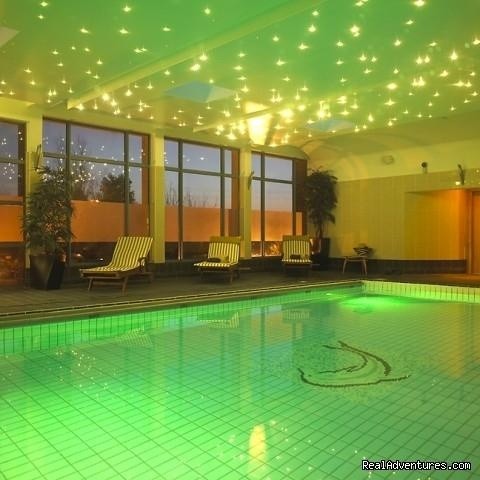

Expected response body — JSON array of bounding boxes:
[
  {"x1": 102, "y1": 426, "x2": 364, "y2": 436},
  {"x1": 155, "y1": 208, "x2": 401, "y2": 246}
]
[{"x1": 421, "y1": 161, "x2": 427, "y2": 168}]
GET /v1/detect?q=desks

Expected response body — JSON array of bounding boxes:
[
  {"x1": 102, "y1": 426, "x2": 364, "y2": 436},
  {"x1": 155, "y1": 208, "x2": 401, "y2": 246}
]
[{"x1": 343, "y1": 255, "x2": 368, "y2": 276}]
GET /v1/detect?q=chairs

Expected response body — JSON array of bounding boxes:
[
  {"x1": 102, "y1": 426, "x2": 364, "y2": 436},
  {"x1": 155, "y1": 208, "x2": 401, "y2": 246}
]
[
  {"x1": 194, "y1": 236, "x2": 243, "y2": 282},
  {"x1": 282, "y1": 235, "x2": 312, "y2": 275},
  {"x1": 79, "y1": 236, "x2": 154, "y2": 293}
]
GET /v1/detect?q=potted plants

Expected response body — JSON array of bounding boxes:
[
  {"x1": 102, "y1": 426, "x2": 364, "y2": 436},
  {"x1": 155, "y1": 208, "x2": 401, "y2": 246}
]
[
  {"x1": 302, "y1": 166, "x2": 338, "y2": 270},
  {"x1": 19, "y1": 166, "x2": 77, "y2": 290}
]
